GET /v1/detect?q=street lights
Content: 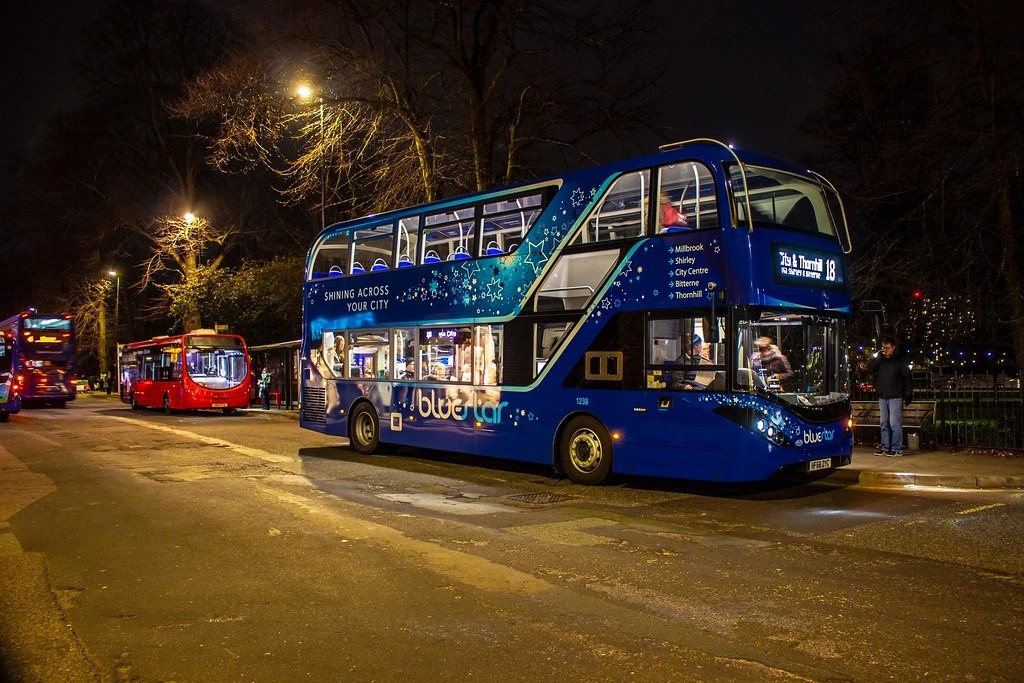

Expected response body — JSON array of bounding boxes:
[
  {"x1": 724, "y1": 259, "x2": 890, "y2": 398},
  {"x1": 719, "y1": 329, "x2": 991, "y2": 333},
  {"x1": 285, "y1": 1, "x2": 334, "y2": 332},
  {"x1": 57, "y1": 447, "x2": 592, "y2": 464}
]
[
  {"x1": 109, "y1": 270, "x2": 120, "y2": 336},
  {"x1": 185, "y1": 210, "x2": 205, "y2": 329},
  {"x1": 298, "y1": 86, "x2": 325, "y2": 231}
]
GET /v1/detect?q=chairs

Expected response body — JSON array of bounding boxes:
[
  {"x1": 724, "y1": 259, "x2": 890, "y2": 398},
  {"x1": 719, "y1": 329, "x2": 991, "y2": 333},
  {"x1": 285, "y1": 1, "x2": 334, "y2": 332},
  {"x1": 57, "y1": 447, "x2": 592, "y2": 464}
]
[{"x1": 328, "y1": 241, "x2": 517, "y2": 278}]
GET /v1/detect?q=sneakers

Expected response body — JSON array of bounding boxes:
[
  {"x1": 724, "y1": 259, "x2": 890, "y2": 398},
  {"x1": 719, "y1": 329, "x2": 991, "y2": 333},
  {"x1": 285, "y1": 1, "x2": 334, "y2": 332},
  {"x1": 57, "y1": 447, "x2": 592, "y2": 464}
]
[
  {"x1": 873, "y1": 450, "x2": 889, "y2": 456},
  {"x1": 887, "y1": 449, "x2": 904, "y2": 456}
]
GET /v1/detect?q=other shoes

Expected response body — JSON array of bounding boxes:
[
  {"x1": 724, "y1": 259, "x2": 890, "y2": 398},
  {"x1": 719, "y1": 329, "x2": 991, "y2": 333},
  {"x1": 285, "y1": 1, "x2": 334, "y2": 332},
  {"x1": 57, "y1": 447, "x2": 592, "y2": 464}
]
[{"x1": 262, "y1": 406, "x2": 270, "y2": 410}]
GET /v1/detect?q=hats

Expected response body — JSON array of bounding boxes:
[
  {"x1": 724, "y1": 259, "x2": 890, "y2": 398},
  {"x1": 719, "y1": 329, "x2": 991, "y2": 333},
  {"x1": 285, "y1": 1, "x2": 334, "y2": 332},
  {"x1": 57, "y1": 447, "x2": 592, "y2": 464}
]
[
  {"x1": 686, "y1": 333, "x2": 701, "y2": 347},
  {"x1": 406, "y1": 357, "x2": 414, "y2": 366}
]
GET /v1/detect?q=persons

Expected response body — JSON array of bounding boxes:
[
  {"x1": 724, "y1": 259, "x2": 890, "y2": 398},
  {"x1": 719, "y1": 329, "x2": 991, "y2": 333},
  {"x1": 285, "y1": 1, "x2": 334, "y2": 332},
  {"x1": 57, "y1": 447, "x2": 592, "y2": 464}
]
[
  {"x1": 645, "y1": 192, "x2": 686, "y2": 230},
  {"x1": 97, "y1": 370, "x2": 114, "y2": 395},
  {"x1": 401, "y1": 341, "x2": 429, "y2": 378},
  {"x1": 333, "y1": 336, "x2": 362, "y2": 378},
  {"x1": 187, "y1": 360, "x2": 199, "y2": 374},
  {"x1": 672, "y1": 335, "x2": 708, "y2": 391},
  {"x1": 867, "y1": 339, "x2": 913, "y2": 456},
  {"x1": 750, "y1": 336, "x2": 795, "y2": 382},
  {"x1": 258, "y1": 367, "x2": 272, "y2": 410}
]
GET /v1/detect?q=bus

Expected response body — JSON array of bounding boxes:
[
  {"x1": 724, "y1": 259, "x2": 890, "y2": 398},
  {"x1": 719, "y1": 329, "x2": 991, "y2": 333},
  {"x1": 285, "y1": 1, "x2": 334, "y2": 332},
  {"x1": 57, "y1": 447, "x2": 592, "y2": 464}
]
[
  {"x1": 120, "y1": 331, "x2": 271, "y2": 414},
  {"x1": 0, "y1": 307, "x2": 79, "y2": 422},
  {"x1": 299, "y1": 138, "x2": 890, "y2": 487}
]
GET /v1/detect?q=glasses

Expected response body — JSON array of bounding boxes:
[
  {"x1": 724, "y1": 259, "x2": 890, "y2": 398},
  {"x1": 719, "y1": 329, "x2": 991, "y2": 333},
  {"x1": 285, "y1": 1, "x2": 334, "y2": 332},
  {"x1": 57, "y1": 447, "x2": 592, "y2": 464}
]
[{"x1": 881, "y1": 345, "x2": 893, "y2": 350}]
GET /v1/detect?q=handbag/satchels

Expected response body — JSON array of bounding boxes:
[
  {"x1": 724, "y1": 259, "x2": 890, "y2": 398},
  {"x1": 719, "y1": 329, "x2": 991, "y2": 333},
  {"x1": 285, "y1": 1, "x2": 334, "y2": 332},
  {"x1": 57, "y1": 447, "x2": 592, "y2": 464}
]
[{"x1": 104, "y1": 381, "x2": 108, "y2": 388}]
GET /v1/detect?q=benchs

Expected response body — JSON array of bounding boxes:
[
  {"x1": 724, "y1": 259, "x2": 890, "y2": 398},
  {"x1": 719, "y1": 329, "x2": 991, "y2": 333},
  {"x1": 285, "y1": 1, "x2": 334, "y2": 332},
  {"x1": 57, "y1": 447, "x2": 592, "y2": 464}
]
[{"x1": 851, "y1": 400, "x2": 938, "y2": 448}]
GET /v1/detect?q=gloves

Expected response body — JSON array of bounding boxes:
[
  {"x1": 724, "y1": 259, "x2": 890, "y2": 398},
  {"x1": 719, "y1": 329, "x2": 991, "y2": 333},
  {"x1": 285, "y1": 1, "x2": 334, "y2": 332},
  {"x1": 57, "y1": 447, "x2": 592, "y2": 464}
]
[
  {"x1": 876, "y1": 350, "x2": 885, "y2": 360},
  {"x1": 904, "y1": 398, "x2": 911, "y2": 407}
]
